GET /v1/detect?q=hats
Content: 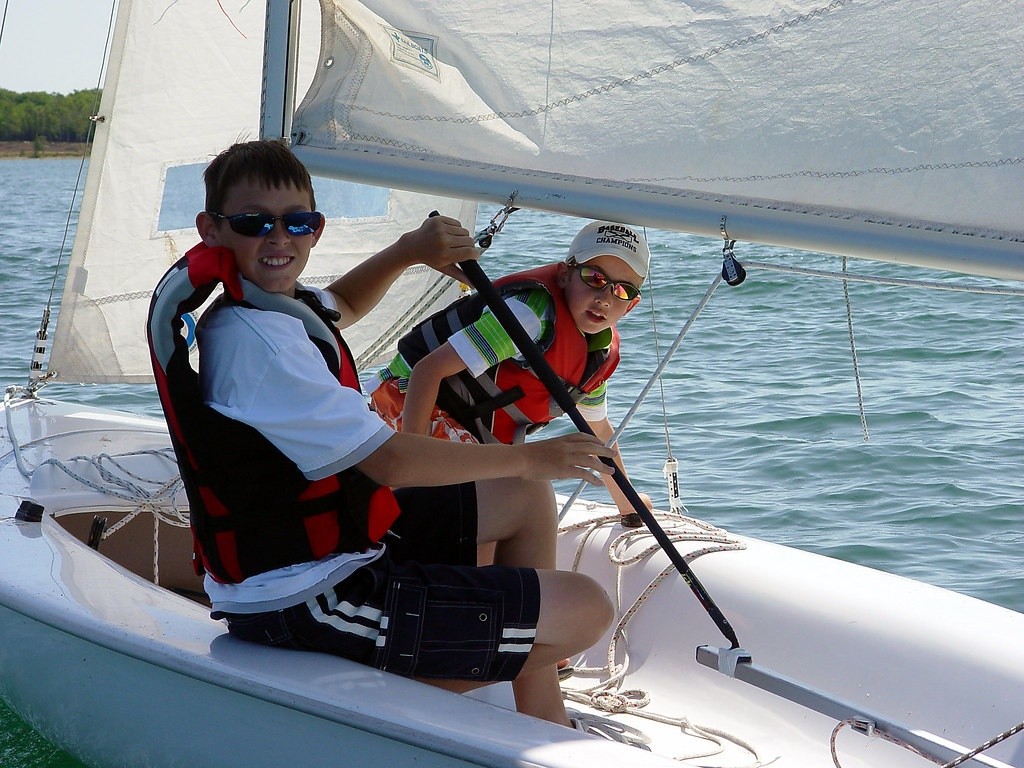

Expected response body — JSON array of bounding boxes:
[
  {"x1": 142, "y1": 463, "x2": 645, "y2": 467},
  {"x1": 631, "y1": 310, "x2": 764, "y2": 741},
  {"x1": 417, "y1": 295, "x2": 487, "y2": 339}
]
[{"x1": 565, "y1": 221, "x2": 651, "y2": 292}]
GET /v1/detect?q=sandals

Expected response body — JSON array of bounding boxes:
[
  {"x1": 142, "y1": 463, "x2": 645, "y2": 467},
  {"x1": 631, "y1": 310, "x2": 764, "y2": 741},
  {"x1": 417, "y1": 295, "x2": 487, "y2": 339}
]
[{"x1": 558, "y1": 666, "x2": 575, "y2": 679}]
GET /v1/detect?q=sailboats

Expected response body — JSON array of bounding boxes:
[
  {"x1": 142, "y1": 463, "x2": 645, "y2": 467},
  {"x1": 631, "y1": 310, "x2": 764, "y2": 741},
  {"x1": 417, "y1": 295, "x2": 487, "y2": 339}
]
[{"x1": 0, "y1": 0, "x2": 1022, "y2": 765}]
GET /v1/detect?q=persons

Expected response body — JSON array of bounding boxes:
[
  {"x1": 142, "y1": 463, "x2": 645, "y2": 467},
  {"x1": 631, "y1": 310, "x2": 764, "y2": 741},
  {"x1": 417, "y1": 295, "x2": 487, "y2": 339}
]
[{"x1": 195, "y1": 141, "x2": 651, "y2": 753}]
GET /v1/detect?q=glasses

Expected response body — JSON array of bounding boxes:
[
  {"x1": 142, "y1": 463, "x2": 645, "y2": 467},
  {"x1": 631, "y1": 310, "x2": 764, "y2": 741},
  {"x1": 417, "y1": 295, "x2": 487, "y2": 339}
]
[
  {"x1": 568, "y1": 262, "x2": 642, "y2": 301},
  {"x1": 206, "y1": 211, "x2": 323, "y2": 238}
]
